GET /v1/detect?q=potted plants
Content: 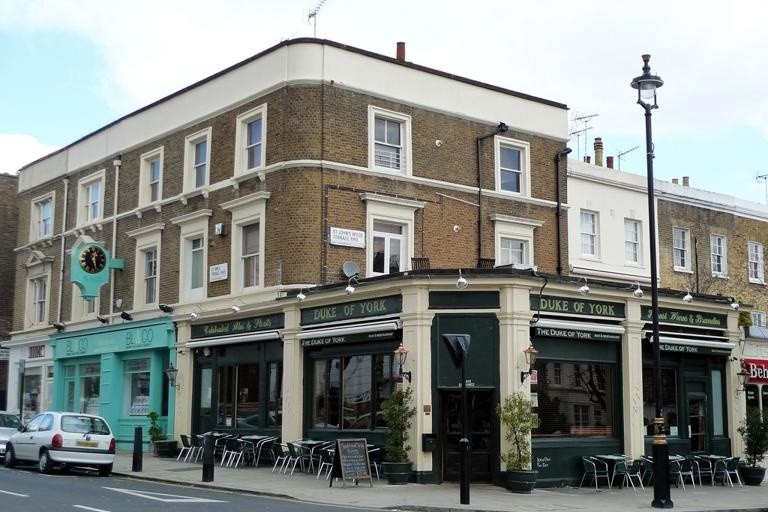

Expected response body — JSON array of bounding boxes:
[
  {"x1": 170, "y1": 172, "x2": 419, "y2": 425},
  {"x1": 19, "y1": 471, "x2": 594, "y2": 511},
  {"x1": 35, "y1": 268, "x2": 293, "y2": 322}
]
[
  {"x1": 736, "y1": 407, "x2": 768, "y2": 486},
  {"x1": 381, "y1": 386, "x2": 417, "y2": 485},
  {"x1": 495, "y1": 392, "x2": 542, "y2": 494},
  {"x1": 146, "y1": 412, "x2": 168, "y2": 457}
]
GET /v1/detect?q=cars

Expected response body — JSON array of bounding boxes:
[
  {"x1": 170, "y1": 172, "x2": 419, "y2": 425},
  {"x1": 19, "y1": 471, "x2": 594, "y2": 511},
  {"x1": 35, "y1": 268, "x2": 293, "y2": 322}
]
[
  {"x1": 0, "y1": 411, "x2": 24, "y2": 466},
  {"x1": 6, "y1": 410, "x2": 116, "y2": 475}
]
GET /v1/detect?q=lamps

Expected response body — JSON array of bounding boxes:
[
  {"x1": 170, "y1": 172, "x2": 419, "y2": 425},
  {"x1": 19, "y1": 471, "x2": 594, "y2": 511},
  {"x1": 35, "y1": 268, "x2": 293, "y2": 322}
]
[
  {"x1": 576, "y1": 276, "x2": 590, "y2": 297},
  {"x1": 683, "y1": 288, "x2": 693, "y2": 303},
  {"x1": 232, "y1": 300, "x2": 241, "y2": 313},
  {"x1": 345, "y1": 275, "x2": 357, "y2": 296},
  {"x1": 190, "y1": 313, "x2": 199, "y2": 321},
  {"x1": 729, "y1": 355, "x2": 750, "y2": 386},
  {"x1": 53, "y1": 305, "x2": 174, "y2": 333},
  {"x1": 730, "y1": 296, "x2": 739, "y2": 309},
  {"x1": 296, "y1": 286, "x2": 312, "y2": 300},
  {"x1": 630, "y1": 282, "x2": 643, "y2": 297},
  {"x1": 394, "y1": 343, "x2": 411, "y2": 383},
  {"x1": 456, "y1": 268, "x2": 468, "y2": 289},
  {"x1": 520, "y1": 344, "x2": 539, "y2": 384},
  {"x1": 166, "y1": 361, "x2": 180, "y2": 388}
]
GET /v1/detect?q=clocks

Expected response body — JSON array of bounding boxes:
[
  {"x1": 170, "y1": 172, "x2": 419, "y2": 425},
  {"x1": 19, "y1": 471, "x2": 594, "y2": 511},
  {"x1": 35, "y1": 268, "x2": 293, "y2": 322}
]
[{"x1": 79, "y1": 244, "x2": 106, "y2": 274}]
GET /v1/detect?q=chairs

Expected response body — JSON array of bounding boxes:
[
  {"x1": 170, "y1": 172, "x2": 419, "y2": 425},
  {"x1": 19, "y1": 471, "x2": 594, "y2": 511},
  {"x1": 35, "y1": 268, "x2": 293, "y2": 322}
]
[
  {"x1": 176, "y1": 431, "x2": 381, "y2": 483},
  {"x1": 578, "y1": 453, "x2": 743, "y2": 491}
]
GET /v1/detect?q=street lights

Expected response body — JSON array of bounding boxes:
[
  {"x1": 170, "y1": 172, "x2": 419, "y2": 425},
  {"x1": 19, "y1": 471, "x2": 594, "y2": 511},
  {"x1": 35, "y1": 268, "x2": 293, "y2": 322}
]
[{"x1": 631, "y1": 53, "x2": 672, "y2": 506}]
